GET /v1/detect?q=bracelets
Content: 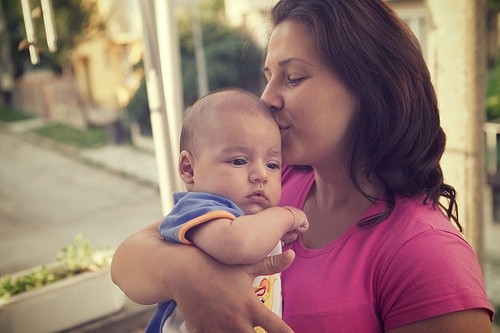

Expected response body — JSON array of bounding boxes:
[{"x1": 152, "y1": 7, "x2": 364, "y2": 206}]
[{"x1": 282, "y1": 206, "x2": 295, "y2": 231}]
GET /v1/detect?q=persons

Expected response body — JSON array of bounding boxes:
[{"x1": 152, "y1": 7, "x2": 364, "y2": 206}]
[
  {"x1": 110, "y1": 0, "x2": 495, "y2": 333},
  {"x1": 144, "y1": 86, "x2": 309, "y2": 333}
]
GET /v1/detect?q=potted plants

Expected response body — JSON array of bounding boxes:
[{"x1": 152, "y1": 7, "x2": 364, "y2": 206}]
[{"x1": 0, "y1": 245, "x2": 126, "y2": 333}]
